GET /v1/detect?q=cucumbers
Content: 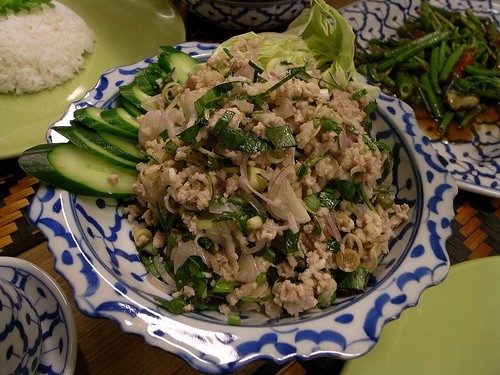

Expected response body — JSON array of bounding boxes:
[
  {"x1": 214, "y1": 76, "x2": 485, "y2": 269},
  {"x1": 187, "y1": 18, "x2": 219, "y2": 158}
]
[{"x1": 18, "y1": 44, "x2": 202, "y2": 198}]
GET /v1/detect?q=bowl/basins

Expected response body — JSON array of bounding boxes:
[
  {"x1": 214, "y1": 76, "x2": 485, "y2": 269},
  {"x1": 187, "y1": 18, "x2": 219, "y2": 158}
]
[
  {"x1": 185, "y1": 0, "x2": 308, "y2": 32},
  {"x1": 0, "y1": 256, "x2": 78, "y2": 372}
]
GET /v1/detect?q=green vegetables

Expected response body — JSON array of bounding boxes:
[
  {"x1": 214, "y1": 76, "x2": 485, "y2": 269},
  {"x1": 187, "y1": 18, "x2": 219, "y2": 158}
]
[
  {"x1": 354, "y1": 0, "x2": 500, "y2": 135},
  {"x1": 0, "y1": 0, "x2": 56, "y2": 17}
]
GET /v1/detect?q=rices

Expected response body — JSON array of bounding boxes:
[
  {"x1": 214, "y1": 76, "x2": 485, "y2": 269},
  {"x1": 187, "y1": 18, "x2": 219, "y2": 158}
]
[{"x1": 0, "y1": 0, "x2": 97, "y2": 95}]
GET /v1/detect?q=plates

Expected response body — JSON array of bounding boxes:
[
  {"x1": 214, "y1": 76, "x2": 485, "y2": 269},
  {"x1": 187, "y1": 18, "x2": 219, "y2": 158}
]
[
  {"x1": 28, "y1": 41, "x2": 458, "y2": 375},
  {"x1": 336, "y1": 255, "x2": 500, "y2": 375},
  {"x1": 0, "y1": 0, "x2": 186, "y2": 161},
  {"x1": 336, "y1": 0, "x2": 500, "y2": 198}
]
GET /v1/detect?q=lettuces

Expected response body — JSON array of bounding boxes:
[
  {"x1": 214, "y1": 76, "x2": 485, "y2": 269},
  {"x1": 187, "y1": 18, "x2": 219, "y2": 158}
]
[{"x1": 206, "y1": 0, "x2": 355, "y2": 88}]
